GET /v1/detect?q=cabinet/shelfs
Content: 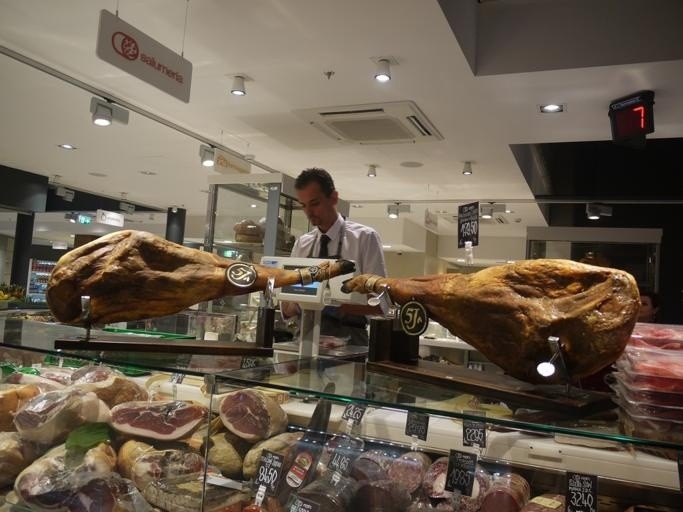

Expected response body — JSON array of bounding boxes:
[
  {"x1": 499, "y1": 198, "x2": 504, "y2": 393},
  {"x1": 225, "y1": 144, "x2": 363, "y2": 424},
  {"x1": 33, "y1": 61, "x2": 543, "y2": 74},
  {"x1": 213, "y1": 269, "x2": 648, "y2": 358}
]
[
  {"x1": 207, "y1": 172, "x2": 355, "y2": 281},
  {"x1": 2, "y1": 312, "x2": 680, "y2": 509}
]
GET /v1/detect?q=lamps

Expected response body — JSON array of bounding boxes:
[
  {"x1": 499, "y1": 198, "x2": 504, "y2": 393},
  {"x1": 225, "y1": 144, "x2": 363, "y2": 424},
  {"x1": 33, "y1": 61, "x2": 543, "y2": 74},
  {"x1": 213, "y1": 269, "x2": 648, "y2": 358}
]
[
  {"x1": 367, "y1": 162, "x2": 378, "y2": 178},
  {"x1": 372, "y1": 56, "x2": 396, "y2": 85},
  {"x1": 91, "y1": 94, "x2": 114, "y2": 133},
  {"x1": 585, "y1": 203, "x2": 605, "y2": 224},
  {"x1": 529, "y1": 332, "x2": 590, "y2": 402},
  {"x1": 383, "y1": 204, "x2": 404, "y2": 221},
  {"x1": 170, "y1": 204, "x2": 179, "y2": 215},
  {"x1": 479, "y1": 207, "x2": 496, "y2": 224},
  {"x1": 230, "y1": 75, "x2": 248, "y2": 97},
  {"x1": 196, "y1": 149, "x2": 217, "y2": 172},
  {"x1": 463, "y1": 161, "x2": 473, "y2": 176}
]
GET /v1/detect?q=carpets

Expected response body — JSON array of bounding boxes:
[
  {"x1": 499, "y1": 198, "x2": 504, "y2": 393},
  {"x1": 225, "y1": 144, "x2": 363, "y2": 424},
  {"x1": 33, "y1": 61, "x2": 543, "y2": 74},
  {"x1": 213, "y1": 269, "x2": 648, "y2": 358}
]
[{"x1": 318, "y1": 235, "x2": 331, "y2": 258}]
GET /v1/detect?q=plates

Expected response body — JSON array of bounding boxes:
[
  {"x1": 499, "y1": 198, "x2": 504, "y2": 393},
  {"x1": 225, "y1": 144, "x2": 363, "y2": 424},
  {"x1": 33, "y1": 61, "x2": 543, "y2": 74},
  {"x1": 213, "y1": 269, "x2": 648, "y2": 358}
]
[{"x1": 207, "y1": 172, "x2": 355, "y2": 281}]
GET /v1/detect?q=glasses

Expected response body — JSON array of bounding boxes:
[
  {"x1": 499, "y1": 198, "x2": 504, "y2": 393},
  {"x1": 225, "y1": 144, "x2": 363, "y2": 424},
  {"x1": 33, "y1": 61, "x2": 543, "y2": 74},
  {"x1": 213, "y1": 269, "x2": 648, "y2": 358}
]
[
  {"x1": 91, "y1": 94, "x2": 114, "y2": 133},
  {"x1": 479, "y1": 207, "x2": 496, "y2": 224},
  {"x1": 170, "y1": 204, "x2": 179, "y2": 215},
  {"x1": 230, "y1": 75, "x2": 248, "y2": 97},
  {"x1": 367, "y1": 162, "x2": 378, "y2": 178},
  {"x1": 529, "y1": 332, "x2": 590, "y2": 402},
  {"x1": 372, "y1": 56, "x2": 396, "y2": 85},
  {"x1": 196, "y1": 149, "x2": 217, "y2": 172},
  {"x1": 585, "y1": 203, "x2": 605, "y2": 224},
  {"x1": 463, "y1": 161, "x2": 473, "y2": 176},
  {"x1": 383, "y1": 204, "x2": 404, "y2": 221}
]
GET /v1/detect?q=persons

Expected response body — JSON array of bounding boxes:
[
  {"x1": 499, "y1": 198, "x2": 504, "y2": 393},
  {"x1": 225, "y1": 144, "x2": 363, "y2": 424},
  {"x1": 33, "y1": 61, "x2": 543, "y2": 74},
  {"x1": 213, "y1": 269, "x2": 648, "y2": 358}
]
[
  {"x1": 637, "y1": 289, "x2": 663, "y2": 324},
  {"x1": 278, "y1": 167, "x2": 392, "y2": 372}
]
